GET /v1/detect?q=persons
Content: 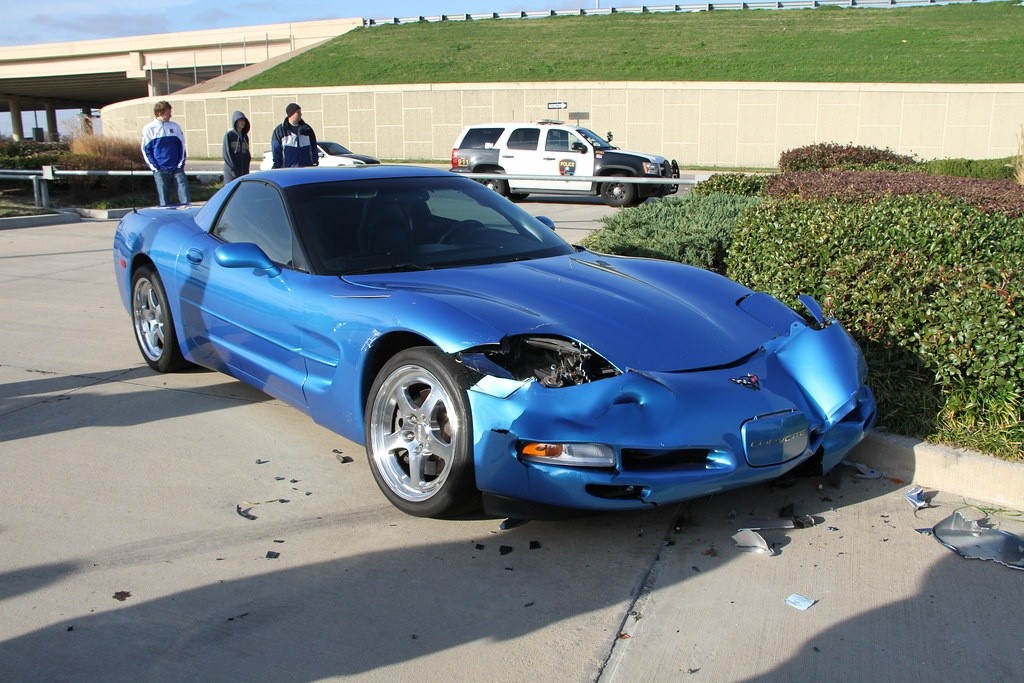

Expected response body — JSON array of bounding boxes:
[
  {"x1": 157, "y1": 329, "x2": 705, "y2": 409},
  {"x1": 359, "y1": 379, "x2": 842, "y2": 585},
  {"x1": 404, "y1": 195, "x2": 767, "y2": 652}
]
[
  {"x1": 141, "y1": 101, "x2": 190, "y2": 205},
  {"x1": 271, "y1": 103, "x2": 319, "y2": 168},
  {"x1": 222, "y1": 111, "x2": 251, "y2": 184}
]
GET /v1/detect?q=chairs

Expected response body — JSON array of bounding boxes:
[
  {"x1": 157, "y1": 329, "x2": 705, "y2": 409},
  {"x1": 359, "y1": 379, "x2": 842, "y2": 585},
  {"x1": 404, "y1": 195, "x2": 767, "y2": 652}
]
[{"x1": 384, "y1": 192, "x2": 447, "y2": 267}]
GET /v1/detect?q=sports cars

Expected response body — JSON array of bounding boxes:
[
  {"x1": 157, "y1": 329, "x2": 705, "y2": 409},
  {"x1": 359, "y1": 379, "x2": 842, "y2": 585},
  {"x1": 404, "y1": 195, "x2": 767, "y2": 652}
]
[{"x1": 111, "y1": 163, "x2": 876, "y2": 520}]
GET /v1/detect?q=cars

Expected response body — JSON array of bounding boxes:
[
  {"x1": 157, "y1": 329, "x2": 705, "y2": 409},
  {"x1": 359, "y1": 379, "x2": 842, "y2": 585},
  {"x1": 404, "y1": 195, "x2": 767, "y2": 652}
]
[{"x1": 259, "y1": 140, "x2": 380, "y2": 167}]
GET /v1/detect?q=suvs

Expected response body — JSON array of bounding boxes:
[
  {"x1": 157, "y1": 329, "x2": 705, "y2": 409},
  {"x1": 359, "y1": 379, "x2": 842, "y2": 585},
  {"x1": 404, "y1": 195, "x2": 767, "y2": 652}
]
[{"x1": 449, "y1": 119, "x2": 682, "y2": 206}]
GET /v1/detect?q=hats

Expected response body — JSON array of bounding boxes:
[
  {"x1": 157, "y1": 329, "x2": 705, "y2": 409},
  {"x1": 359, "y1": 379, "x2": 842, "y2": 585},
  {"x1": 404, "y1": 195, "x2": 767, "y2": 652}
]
[{"x1": 286, "y1": 103, "x2": 300, "y2": 118}]
